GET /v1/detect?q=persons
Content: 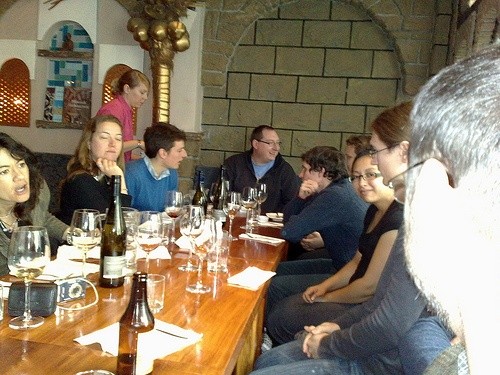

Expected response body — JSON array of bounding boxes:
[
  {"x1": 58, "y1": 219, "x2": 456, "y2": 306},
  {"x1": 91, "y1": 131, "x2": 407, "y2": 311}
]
[
  {"x1": 95, "y1": 69, "x2": 150, "y2": 163},
  {"x1": 266, "y1": 137, "x2": 373, "y2": 306},
  {"x1": 268, "y1": 149, "x2": 404, "y2": 346},
  {"x1": 224, "y1": 125, "x2": 296, "y2": 215},
  {"x1": 344, "y1": 135, "x2": 375, "y2": 171},
  {"x1": 404, "y1": 39, "x2": 500, "y2": 375},
  {"x1": 123, "y1": 122, "x2": 187, "y2": 222},
  {"x1": 247, "y1": 101, "x2": 454, "y2": 375},
  {"x1": 60, "y1": 115, "x2": 132, "y2": 227},
  {"x1": 0, "y1": 132, "x2": 71, "y2": 276}
]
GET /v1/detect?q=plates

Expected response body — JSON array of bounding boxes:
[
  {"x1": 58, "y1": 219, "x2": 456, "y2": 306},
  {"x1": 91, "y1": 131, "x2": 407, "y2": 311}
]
[{"x1": 266, "y1": 213, "x2": 283, "y2": 222}]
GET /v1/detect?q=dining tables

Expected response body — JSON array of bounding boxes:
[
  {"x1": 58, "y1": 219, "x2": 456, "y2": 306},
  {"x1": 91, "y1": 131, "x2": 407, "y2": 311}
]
[{"x1": 0, "y1": 210, "x2": 288, "y2": 375}]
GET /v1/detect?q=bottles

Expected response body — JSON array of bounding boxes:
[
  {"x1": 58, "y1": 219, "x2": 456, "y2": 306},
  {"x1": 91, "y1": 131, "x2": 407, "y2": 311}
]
[
  {"x1": 212, "y1": 167, "x2": 228, "y2": 227},
  {"x1": 99, "y1": 175, "x2": 127, "y2": 289},
  {"x1": 116, "y1": 271, "x2": 156, "y2": 375},
  {"x1": 192, "y1": 170, "x2": 207, "y2": 216}
]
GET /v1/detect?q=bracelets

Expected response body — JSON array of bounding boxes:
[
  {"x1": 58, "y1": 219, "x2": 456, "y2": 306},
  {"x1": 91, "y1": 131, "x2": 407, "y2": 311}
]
[{"x1": 137, "y1": 140, "x2": 141, "y2": 148}]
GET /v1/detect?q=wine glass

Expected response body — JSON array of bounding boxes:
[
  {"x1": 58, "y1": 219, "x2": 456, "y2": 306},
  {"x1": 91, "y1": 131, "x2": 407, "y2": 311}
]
[
  {"x1": 68, "y1": 208, "x2": 102, "y2": 278},
  {"x1": 134, "y1": 210, "x2": 163, "y2": 274},
  {"x1": 177, "y1": 181, "x2": 269, "y2": 293},
  {"x1": 165, "y1": 191, "x2": 182, "y2": 245},
  {"x1": 7, "y1": 225, "x2": 51, "y2": 330}
]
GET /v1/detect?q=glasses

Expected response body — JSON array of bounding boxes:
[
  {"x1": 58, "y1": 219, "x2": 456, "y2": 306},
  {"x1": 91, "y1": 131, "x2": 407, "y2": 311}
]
[
  {"x1": 255, "y1": 139, "x2": 282, "y2": 147},
  {"x1": 368, "y1": 144, "x2": 401, "y2": 158},
  {"x1": 348, "y1": 171, "x2": 381, "y2": 183},
  {"x1": 388, "y1": 161, "x2": 455, "y2": 204}
]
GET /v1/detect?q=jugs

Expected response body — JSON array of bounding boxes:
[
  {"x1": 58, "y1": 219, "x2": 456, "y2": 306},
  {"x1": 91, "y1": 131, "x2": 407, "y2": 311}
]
[{"x1": 94, "y1": 207, "x2": 141, "y2": 266}]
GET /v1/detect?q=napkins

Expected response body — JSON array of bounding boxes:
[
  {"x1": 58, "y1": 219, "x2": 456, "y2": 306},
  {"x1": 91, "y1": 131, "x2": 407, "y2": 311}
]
[
  {"x1": 239, "y1": 233, "x2": 285, "y2": 246},
  {"x1": 73, "y1": 317, "x2": 203, "y2": 361},
  {"x1": 227, "y1": 265, "x2": 277, "y2": 291},
  {"x1": 174, "y1": 229, "x2": 213, "y2": 250},
  {"x1": 256, "y1": 221, "x2": 284, "y2": 228},
  {"x1": 34, "y1": 258, "x2": 100, "y2": 285},
  {"x1": 57, "y1": 243, "x2": 101, "y2": 259},
  {"x1": 125, "y1": 244, "x2": 172, "y2": 260}
]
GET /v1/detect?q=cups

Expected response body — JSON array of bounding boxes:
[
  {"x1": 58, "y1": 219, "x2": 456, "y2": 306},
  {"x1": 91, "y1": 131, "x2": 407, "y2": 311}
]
[
  {"x1": 146, "y1": 274, "x2": 165, "y2": 313},
  {"x1": 246, "y1": 210, "x2": 258, "y2": 234},
  {"x1": 207, "y1": 229, "x2": 230, "y2": 274},
  {"x1": 0, "y1": 283, "x2": 4, "y2": 322}
]
